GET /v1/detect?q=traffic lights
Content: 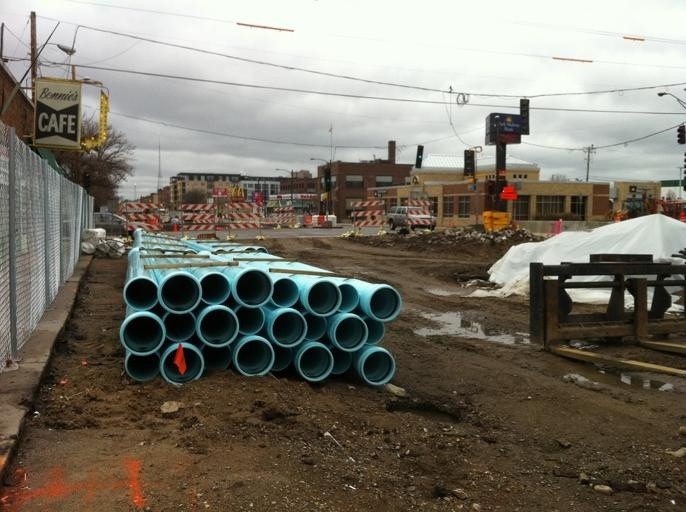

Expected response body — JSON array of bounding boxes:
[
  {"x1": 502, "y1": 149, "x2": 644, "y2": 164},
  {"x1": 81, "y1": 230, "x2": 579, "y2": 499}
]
[
  {"x1": 521, "y1": 99, "x2": 529, "y2": 135},
  {"x1": 677, "y1": 124, "x2": 686, "y2": 145},
  {"x1": 488, "y1": 181, "x2": 494, "y2": 195},
  {"x1": 416, "y1": 146, "x2": 424, "y2": 168}
]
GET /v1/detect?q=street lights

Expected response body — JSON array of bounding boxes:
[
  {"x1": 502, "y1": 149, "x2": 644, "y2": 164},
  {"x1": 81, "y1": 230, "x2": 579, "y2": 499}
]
[
  {"x1": 275, "y1": 168, "x2": 294, "y2": 201},
  {"x1": 36, "y1": 41, "x2": 77, "y2": 63},
  {"x1": 657, "y1": 92, "x2": 686, "y2": 109},
  {"x1": 310, "y1": 158, "x2": 331, "y2": 213}
]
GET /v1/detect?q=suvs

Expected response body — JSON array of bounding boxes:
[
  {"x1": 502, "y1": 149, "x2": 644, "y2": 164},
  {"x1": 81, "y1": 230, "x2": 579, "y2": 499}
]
[{"x1": 387, "y1": 206, "x2": 436, "y2": 232}]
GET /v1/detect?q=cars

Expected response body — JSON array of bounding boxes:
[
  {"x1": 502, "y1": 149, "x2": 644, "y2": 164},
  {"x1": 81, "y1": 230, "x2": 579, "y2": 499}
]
[{"x1": 92, "y1": 211, "x2": 129, "y2": 235}]
